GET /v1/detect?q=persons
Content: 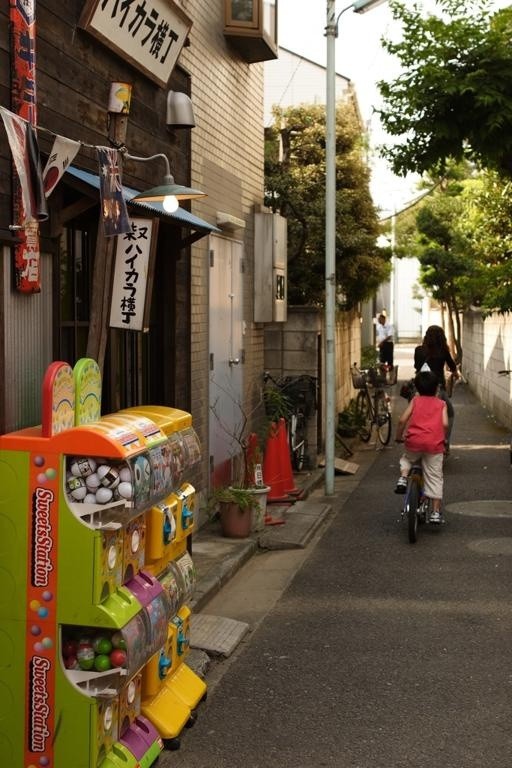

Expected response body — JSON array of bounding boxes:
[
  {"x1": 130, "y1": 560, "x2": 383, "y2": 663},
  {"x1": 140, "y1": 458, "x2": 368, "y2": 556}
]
[
  {"x1": 376, "y1": 314, "x2": 394, "y2": 380},
  {"x1": 397, "y1": 325, "x2": 458, "y2": 522}
]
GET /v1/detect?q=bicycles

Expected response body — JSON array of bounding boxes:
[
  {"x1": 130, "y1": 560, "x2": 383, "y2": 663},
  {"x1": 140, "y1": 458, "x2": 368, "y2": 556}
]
[
  {"x1": 262, "y1": 370, "x2": 319, "y2": 472},
  {"x1": 351, "y1": 362, "x2": 392, "y2": 445},
  {"x1": 395, "y1": 440, "x2": 449, "y2": 544}
]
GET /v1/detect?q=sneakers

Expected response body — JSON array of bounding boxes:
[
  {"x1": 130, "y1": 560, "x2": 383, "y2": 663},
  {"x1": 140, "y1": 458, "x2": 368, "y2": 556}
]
[
  {"x1": 428, "y1": 510, "x2": 446, "y2": 524},
  {"x1": 395, "y1": 476, "x2": 408, "y2": 493}
]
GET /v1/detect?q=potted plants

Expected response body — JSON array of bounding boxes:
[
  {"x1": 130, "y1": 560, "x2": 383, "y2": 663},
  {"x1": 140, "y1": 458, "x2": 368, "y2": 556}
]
[{"x1": 195, "y1": 368, "x2": 305, "y2": 538}]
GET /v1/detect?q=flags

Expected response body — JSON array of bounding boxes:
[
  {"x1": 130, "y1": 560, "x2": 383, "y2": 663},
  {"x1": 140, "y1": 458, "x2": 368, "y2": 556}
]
[
  {"x1": 96, "y1": 145, "x2": 132, "y2": 236},
  {"x1": 0, "y1": 103, "x2": 81, "y2": 223}
]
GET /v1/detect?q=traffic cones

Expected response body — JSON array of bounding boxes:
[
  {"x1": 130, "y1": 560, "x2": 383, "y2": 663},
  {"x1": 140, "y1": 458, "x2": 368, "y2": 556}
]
[{"x1": 246, "y1": 417, "x2": 298, "y2": 501}]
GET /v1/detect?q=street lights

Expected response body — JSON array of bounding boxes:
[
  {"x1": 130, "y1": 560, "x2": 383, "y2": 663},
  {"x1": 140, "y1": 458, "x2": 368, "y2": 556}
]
[{"x1": 324, "y1": 0, "x2": 390, "y2": 496}]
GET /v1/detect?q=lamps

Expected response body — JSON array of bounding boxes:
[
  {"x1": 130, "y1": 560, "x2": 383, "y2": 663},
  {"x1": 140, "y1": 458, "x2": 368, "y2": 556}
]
[{"x1": 116, "y1": 145, "x2": 207, "y2": 214}]
